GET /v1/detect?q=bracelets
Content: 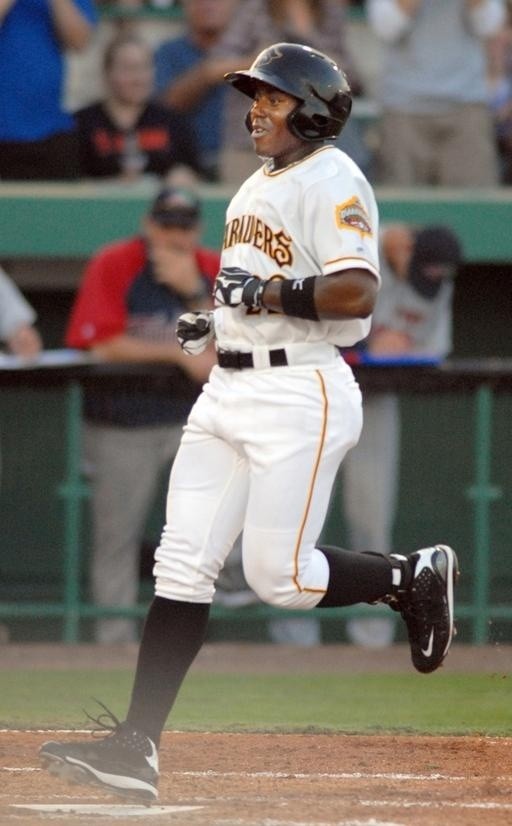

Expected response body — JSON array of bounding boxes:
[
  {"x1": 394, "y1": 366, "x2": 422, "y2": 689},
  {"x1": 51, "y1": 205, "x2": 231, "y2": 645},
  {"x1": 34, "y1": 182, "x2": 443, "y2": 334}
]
[{"x1": 278, "y1": 274, "x2": 322, "y2": 324}]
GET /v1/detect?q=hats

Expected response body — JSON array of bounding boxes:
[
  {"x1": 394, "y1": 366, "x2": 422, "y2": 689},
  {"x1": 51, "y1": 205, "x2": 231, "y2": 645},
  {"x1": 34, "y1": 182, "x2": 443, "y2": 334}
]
[
  {"x1": 153, "y1": 188, "x2": 201, "y2": 229},
  {"x1": 406, "y1": 226, "x2": 462, "y2": 299}
]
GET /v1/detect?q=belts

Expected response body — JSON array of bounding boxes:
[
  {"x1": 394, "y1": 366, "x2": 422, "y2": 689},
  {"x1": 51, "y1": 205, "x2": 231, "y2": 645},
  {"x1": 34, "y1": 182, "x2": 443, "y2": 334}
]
[{"x1": 217, "y1": 349, "x2": 287, "y2": 368}]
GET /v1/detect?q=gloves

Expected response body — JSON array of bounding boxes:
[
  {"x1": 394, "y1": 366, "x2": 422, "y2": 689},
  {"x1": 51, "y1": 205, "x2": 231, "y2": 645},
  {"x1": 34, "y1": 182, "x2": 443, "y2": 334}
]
[
  {"x1": 213, "y1": 268, "x2": 270, "y2": 309},
  {"x1": 175, "y1": 308, "x2": 214, "y2": 356}
]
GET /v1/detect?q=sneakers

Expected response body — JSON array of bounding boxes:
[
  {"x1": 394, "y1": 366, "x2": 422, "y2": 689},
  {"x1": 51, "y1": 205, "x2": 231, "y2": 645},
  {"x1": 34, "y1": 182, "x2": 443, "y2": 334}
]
[
  {"x1": 36, "y1": 725, "x2": 159, "y2": 802},
  {"x1": 390, "y1": 544, "x2": 459, "y2": 673}
]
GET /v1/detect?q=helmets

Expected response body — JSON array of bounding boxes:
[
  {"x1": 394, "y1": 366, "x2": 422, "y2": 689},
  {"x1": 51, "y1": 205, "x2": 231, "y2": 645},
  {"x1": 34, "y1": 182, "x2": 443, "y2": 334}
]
[{"x1": 224, "y1": 42, "x2": 352, "y2": 141}]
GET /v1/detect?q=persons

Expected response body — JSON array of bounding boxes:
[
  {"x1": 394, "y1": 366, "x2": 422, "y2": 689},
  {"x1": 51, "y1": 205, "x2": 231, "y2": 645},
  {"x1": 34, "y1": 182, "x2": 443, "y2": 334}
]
[
  {"x1": 38, "y1": 40, "x2": 460, "y2": 800},
  {"x1": 1, "y1": 0, "x2": 512, "y2": 187},
  {"x1": 268, "y1": 222, "x2": 464, "y2": 648},
  {"x1": 66, "y1": 188, "x2": 257, "y2": 647},
  {"x1": 0, "y1": 264, "x2": 46, "y2": 358}
]
